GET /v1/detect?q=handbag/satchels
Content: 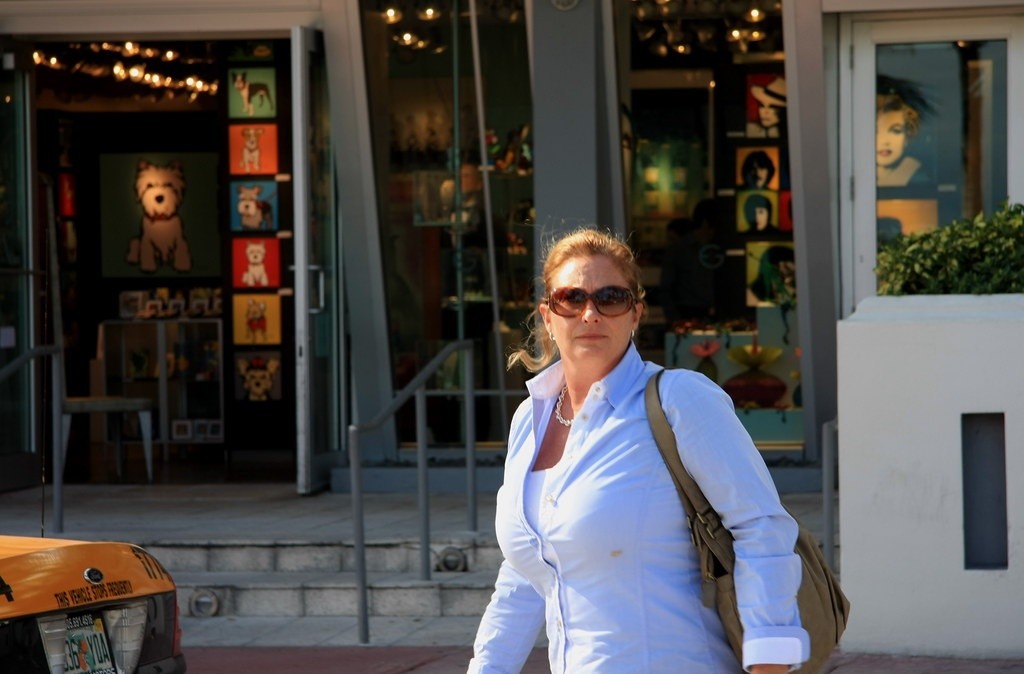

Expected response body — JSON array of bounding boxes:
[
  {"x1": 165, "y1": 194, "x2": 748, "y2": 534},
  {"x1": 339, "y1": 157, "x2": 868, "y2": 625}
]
[{"x1": 645, "y1": 368, "x2": 850, "y2": 674}]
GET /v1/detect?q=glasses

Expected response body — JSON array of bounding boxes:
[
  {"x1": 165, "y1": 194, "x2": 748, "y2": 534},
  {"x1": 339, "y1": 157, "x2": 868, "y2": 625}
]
[{"x1": 543, "y1": 284, "x2": 636, "y2": 318}]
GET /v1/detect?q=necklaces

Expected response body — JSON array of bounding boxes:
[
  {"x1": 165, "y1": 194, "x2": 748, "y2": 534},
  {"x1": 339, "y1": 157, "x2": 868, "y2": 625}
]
[{"x1": 554, "y1": 385, "x2": 575, "y2": 427}]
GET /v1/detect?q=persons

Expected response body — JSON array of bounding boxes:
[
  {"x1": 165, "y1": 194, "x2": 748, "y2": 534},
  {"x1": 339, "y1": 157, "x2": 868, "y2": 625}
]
[{"x1": 466, "y1": 231, "x2": 810, "y2": 674}]
